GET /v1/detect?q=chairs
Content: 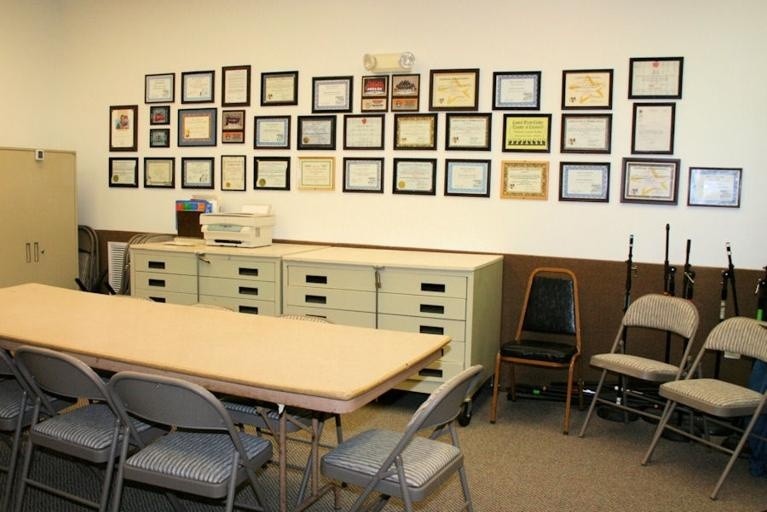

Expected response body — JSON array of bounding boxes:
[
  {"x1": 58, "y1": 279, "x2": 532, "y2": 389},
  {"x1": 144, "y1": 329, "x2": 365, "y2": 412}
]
[
  {"x1": 643, "y1": 315, "x2": 767, "y2": 500},
  {"x1": 320, "y1": 363, "x2": 483, "y2": 512},
  {"x1": 578, "y1": 293, "x2": 702, "y2": 448},
  {"x1": 488, "y1": 266, "x2": 584, "y2": 433},
  {"x1": 108, "y1": 371, "x2": 273, "y2": 512},
  {"x1": 0, "y1": 349, "x2": 79, "y2": 512},
  {"x1": 12, "y1": 346, "x2": 163, "y2": 511},
  {"x1": 219, "y1": 315, "x2": 344, "y2": 512}
]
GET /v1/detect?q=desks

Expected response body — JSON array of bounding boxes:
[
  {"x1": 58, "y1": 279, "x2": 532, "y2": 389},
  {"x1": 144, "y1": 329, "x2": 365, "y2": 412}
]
[{"x1": 0, "y1": 283, "x2": 451, "y2": 511}]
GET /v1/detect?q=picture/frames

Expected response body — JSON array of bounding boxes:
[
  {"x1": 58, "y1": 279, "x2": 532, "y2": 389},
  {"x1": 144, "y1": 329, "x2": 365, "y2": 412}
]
[
  {"x1": 687, "y1": 165, "x2": 743, "y2": 209},
  {"x1": 391, "y1": 97, "x2": 418, "y2": 112},
  {"x1": 311, "y1": 75, "x2": 353, "y2": 113},
  {"x1": 254, "y1": 115, "x2": 290, "y2": 149},
  {"x1": 393, "y1": 157, "x2": 436, "y2": 196},
  {"x1": 631, "y1": 102, "x2": 676, "y2": 154},
  {"x1": 260, "y1": 70, "x2": 298, "y2": 106},
  {"x1": 253, "y1": 156, "x2": 290, "y2": 191},
  {"x1": 150, "y1": 129, "x2": 169, "y2": 147},
  {"x1": 296, "y1": 114, "x2": 337, "y2": 151},
  {"x1": 109, "y1": 157, "x2": 138, "y2": 187},
  {"x1": 221, "y1": 65, "x2": 251, "y2": 107},
  {"x1": 561, "y1": 68, "x2": 614, "y2": 110},
  {"x1": 221, "y1": 155, "x2": 246, "y2": 191},
  {"x1": 343, "y1": 114, "x2": 384, "y2": 150},
  {"x1": 444, "y1": 159, "x2": 491, "y2": 198},
  {"x1": 149, "y1": 106, "x2": 170, "y2": 125},
  {"x1": 222, "y1": 110, "x2": 244, "y2": 143},
  {"x1": 342, "y1": 157, "x2": 383, "y2": 193},
  {"x1": 428, "y1": 68, "x2": 479, "y2": 112},
  {"x1": 392, "y1": 74, "x2": 420, "y2": 97},
  {"x1": 620, "y1": 156, "x2": 681, "y2": 206},
  {"x1": 144, "y1": 156, "x2": 175, "y2": 188},
  {"x1": 145, "y1": 73, "x2": 174, "y2": 103},
  {"x1": 362, "y1": 75, "x2": 389, "y2": 97},
  {"x1": 296, "y1": 155, "x2": 336, "y2": 191},
  {"x1": 499, "y1": 159, "x2": 550, "y2": 201},
  {"x1": 628, "y1": 57, "x2": 683, "y2": 99},
  {"x1": 559, "y1": 161, "x2": 609, "y2": 202},
  {"x1": 109, "y1": 105, "x2": 137, "y2": 152},
  {"x1": 181, "y1": 156, "x2": 214, "y2": 190},
  {"x1": 177, "y1": 107, "x2": 217, "y2": 147},
  {"x1": 394, "y1": 112, "x2": 438, "y2": 150},
  {"x1": 501, "y1": 113, "x2": 552, "y2": 153},
  {"x1": 492, "y1": 70, "x2": 540, "y2": 110},
  {"x1": 361, "y1": 97, "x2": 389, "y2": 112},
  {"x1": 560, "y1": 112, "x2": 612, "y2": 154},
  {"x1": 181, "y1": 70, "x2": 215, "y2": 104},
  {"x1": 445, "y1": 112, "x2": 491, "y2": 151}
]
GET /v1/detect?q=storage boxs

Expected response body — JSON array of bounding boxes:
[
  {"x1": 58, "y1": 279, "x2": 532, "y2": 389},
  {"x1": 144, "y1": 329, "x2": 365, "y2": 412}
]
[{"x1": 196, "y1": 210, "x2": 275, "y2": 248}]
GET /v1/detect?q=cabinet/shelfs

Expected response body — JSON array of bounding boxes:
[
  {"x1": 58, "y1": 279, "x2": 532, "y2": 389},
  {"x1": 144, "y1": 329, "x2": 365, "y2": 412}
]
[
  {"x1": 128, "y1": 239, "x2": 199, "y2": 307},
  {"x1": 201, "y1": 243, "x2": 331, "y2": 322},
  {"x1": 283, "y1": 248, "x2": 503, "y2": 426},
  {"x1": 0, "y1": 146, "x2": 78, "y2": 291}
]
